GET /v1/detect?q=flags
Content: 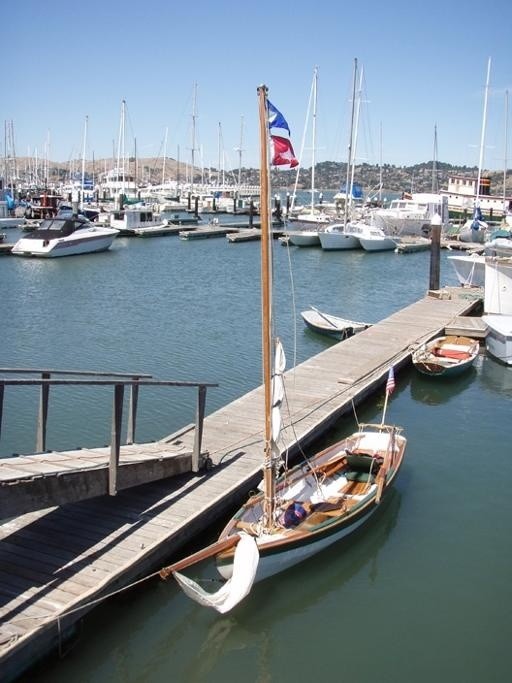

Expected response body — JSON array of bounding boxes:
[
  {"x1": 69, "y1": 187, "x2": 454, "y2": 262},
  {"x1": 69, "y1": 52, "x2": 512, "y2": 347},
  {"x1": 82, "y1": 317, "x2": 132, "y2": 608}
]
[
  {"x1": 269, "y1": 135, "x2": 299, "y2": 170},
  {"x1": 264, "y1": 100, "x2": 291, "y2": 135}
]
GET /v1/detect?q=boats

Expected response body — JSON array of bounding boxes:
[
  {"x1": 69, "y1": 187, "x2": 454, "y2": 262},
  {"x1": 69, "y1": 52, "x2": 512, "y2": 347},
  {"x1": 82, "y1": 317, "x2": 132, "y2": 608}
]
[
  {"x1": 299, "y1": 308, "x2": 377, "y2": 342},
  {"x1": 8, "y1": 202, "x2": 121, "y2": 259},
  {"x1": 439, "y1": 172, "x2": 512, "y2": 224},
  {"x1": 445, "y1": 253, "x2": 512, "y2": 366},
  {"x1": 409, "y1": 334, "x2": 482, "y2": 380}
]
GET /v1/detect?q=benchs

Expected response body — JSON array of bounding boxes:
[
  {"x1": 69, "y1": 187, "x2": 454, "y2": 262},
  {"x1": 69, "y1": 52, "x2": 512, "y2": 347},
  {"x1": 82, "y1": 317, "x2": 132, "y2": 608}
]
[{"x1": 344, "y1": 456, "x2": 379, "y2": 483}]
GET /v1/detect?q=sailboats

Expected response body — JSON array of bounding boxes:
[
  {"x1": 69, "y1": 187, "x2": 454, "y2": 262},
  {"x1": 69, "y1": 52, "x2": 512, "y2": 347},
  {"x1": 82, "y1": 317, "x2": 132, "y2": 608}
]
[
  {"x1": 160, "y1": 82, "x2": 410, "y2": 612},
  {"x1": 456, "y1": 54, "x2": 490, "y2": 243},
  {"x1": 0, "y1": 79, "x2": 260, "y2": 237},
  {"x1": 482, "y1": 87, "x2": 512, "y2": 259},
  {"x1": 282, "y1": 56, "x2": 449, "y2": 253}
]
